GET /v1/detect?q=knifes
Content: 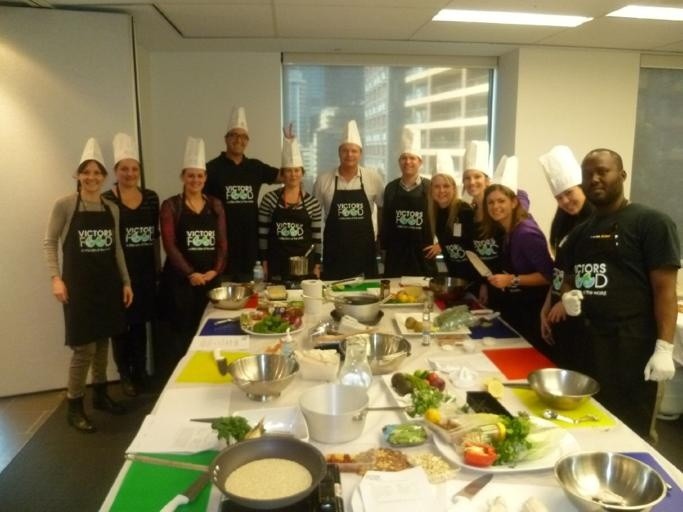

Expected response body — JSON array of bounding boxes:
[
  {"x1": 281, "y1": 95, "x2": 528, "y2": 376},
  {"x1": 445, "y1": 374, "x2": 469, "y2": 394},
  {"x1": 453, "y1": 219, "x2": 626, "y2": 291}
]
[
  {"x1": 451, "y1": 473, "x2": 495, "y2": 511},
  {"x1": 159, "y1": 470, "x2": 213, "y2": 512},
  {"x1": 465, "y1": 250, "x2": 493, "y2": 278}
]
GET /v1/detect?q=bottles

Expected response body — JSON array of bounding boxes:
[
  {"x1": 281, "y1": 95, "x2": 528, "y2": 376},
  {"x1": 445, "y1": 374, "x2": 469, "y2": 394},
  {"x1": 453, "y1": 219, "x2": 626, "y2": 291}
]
[
  {"x1": 252, "y1": 260, "x2": 265, "y2": 294},
  {"x1": 337, "y1": 335, "x2": 374, "y2": 391}
]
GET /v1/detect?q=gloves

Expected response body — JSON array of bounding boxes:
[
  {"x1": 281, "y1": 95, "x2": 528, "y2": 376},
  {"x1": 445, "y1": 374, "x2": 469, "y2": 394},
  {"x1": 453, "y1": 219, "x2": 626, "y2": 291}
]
[
  {"x1": 561, "y1": 290, "x2": 584, "y2": 317},
  {"x1": 645, "y1": 340, "x2": 676, "y2": 382}
]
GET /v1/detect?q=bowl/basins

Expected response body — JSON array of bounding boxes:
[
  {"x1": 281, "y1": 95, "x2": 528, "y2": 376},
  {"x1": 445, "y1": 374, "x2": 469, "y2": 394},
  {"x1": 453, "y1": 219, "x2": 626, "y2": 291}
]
[
  {"x1": 300, "y1": 381, "x2": 369, "y2": 442},
  {"x1": 529, "y1": 364, "x2": 598, "y2": 408},
  {"x1": 553, "y1": 450, "x2": 668, "y2": 512},
  {"x1": 202, "y1": 271, "x2": 472, "y2": 370},
  {"x1": 228, "y1": 353, "x2": 300, "y2": 401}
]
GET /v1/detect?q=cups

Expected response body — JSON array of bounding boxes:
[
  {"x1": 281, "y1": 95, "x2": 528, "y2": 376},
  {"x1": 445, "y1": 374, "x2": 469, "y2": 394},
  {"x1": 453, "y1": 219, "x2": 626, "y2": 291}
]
[{"x1": 289, "y1": 256, "x2": 309, "y2": 276}]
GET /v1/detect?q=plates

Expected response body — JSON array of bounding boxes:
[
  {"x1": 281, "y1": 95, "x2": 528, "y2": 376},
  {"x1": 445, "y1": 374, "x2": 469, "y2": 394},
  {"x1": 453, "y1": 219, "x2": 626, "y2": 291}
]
[
  {"x1": 232, "y1": 404, "x2": 309, "y2": 445},
  {"x1": 384, "y1": 372, "x2": 460, "y2": 422},
  {"x1": 209, "y1": 436, "x2": 326, "y2": 506},
  {"x1": 434, "y1": 418, "x2": 579, "y2": 471}
]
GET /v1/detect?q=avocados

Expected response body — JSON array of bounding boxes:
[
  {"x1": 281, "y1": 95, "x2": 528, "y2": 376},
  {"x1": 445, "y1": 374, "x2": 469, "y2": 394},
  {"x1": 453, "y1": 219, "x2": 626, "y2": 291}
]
[{"x1": 391, "y1": 372, "x2": 412, "y2": 394}]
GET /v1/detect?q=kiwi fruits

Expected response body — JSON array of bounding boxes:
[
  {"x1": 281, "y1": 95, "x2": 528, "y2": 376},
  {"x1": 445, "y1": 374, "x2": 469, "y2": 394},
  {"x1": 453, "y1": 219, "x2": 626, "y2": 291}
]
[{"x1": 406, "y1": 316, "x2": 423, "y2": 332}]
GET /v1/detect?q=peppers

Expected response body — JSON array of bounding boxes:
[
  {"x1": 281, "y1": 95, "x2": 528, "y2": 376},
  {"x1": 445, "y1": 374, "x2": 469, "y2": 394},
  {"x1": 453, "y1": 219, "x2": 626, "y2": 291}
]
[{"x1": 464, "y1": 441, "x2": 497, "y2": 466}]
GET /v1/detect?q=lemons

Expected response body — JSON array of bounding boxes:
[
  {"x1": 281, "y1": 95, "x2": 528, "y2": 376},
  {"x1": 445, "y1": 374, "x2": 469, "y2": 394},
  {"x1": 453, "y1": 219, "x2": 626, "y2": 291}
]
[{"x1": 425, "y1": 408, "x2": 441, "y2": 423}]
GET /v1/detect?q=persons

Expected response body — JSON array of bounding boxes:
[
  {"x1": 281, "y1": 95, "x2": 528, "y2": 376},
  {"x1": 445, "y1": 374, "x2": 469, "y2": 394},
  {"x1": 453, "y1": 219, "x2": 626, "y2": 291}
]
[
  {"x1": 100, "y1": 156, "x2": 161, "y2": 397},
  {"x1": 380, "y1": 124, "x2": 443, "y2": 278},
  {"x1": 538, "y1": 183, "x2": 597, "y2": 359},
  {"x1": 42, "y1": 156, "x2": 136, "y2": 433},
  {"x1": 206, "y1": 124, "x2": 295, "y2": 280},
  {"x1": 428, "y1": 139, "x2": 552, "y2": 328},
  {"x1": 259, "y1": 137, "x2": 322, "y2": 289},
  {"x1": 548, "y1": 148, "x2": 681, "y2": 441},
  {"x1": 312, "y1": 120, "x2": 385, "y2": 281},
  {"x1": 160, "y1": 162, "x2": 228, "y2": 367}
]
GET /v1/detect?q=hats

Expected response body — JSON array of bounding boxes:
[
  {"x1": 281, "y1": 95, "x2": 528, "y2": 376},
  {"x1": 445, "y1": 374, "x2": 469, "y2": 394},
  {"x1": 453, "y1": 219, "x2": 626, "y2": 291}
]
[
  {"x1": 113, "y1": 133, "x2": 140, "y2": 167},
  {"x1": 282, "y1": 137, "x2": 304, "y2": 169},
  {"x1": 226, "y1": 106, "x2": 248, "y2": 135},
  {"x1": 464, "y1": 140, "x2": 491, "y2": 176},
  {"x1": 183, "y1": 138, "x2": 207, "y2": 171},
  {"x1": 340, "y1": 120, "x2": 362, "y2": 149},
  {"x1": 80, "y1": 137, "x2": 109, "y2": 173},
  {"x1": 540, "y1": 144, "x2": 583, "y2": 197},
  {"x1": 430, "y1": 149, "x2": 453, "y2": 180},
  {"x1": 490, "y1": 154, "x2": 518, "y2": 196},
  {"x1": 398, "y1": 125, "x2": 422, "y2": 161}
]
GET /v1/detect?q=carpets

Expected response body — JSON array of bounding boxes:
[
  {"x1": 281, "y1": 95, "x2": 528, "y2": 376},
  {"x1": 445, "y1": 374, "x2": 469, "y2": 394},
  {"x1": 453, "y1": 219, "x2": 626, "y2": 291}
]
[{"x1": 0, "y1": 392, "x2": 160, "y2": 512}]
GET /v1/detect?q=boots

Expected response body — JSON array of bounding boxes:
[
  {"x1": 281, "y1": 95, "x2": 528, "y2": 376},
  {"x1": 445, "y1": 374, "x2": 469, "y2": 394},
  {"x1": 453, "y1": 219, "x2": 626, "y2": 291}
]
[
  {"x1": 136, "y1": 358, "x2": 150, "y2": 388},
  {"x1": 119, "y1": 365, "x2": 138, "y2": 396},
  {"x1": 68, "y1": 396, "x2": 96, "y2": 431},
  {"x1": 92, "y1": 383, "x2": 123, "y2": 414}
]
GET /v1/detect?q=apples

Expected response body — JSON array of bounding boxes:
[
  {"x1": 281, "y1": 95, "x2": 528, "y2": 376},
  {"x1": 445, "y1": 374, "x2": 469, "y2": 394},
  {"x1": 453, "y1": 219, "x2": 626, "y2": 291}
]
[{"x1": 426, "y1": 373, "x2": 445, "y2": 391}]
